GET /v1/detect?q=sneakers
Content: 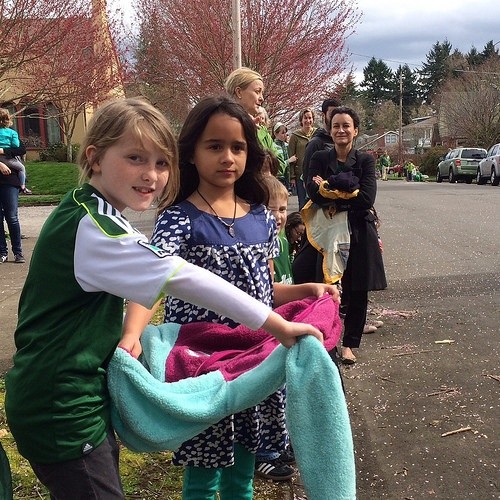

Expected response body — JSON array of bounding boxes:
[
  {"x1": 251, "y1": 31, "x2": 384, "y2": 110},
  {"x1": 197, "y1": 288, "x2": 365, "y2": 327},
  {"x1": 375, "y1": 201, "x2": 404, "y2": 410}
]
[
  {"x1": 363, "y1": 324, "x2": 377, "y2": 333},
  {"x1": 255, "y1": 452, "x2": 296, "y2": 480},
  {"x1": 15, "y1": 253, "x2": 25, "y2": 263},
  {"x1": 19, "y1": 186, "x2": 32, "y2": 194},
  {"x1": 366, "y1": 320, "x2": 383, "y2": 328},
  {"x1": 0, "y1": 254, "x2": 8, "y2": 263}
]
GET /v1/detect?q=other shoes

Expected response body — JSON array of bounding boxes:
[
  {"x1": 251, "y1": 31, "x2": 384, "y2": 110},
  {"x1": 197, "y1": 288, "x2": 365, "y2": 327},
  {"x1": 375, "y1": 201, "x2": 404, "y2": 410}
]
[{"x1": 338, "y1": 346, "x2": 357, "y2": 364}]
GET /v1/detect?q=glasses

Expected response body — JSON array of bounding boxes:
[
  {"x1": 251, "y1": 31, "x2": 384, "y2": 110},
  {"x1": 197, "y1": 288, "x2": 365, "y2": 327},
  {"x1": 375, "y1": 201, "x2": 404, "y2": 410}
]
[{"x1": 294, "y1": 226, "x2": 305, "y2": 237}]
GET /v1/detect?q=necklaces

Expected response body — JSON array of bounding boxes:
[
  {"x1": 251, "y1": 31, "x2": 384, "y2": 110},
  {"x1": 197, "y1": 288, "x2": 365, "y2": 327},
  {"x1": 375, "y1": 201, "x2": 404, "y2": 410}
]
[{"x1": 196, "y1": 188, "x2": 237, "y2": 239}]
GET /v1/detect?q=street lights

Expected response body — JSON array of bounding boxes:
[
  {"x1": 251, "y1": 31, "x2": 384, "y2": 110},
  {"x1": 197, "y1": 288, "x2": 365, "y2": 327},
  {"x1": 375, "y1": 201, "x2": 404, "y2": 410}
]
[{"x1": 397, "y1": 70, "x2": 402, "y2": 164}]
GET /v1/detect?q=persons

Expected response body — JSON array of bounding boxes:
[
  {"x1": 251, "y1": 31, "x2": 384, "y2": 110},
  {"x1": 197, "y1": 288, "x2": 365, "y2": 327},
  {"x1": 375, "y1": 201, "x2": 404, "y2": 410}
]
[
  {"x1": 397, "y1": 158, "x2": 422, "y2": 181},
  {"x1": 375, "y1": 150, "x2": 391, "y2": 181},
  {"x1": 223, "y1": 67, "x2": 389, "y2": 480},
  {"x1": 0, "y1": 107, "x2": 33, "y2": 264},
  {"x1": 5, "y1": 97, "x2": 327, "y2": 499},
  {"x1": 116, "y1": 95, "x2": 340, "y2": 500}
]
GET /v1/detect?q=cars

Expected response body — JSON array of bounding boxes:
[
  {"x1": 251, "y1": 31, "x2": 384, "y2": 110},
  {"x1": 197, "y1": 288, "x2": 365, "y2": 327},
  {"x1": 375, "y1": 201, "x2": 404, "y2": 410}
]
[
  {"x1": 476, "y1": 143, "x2": 500, "y2": 185},
  {"x1": 389, "y1": 164, "x2": 401, "y2": 175},
  {"x1": 436, "y1": 148, "x2": 487, "y2": 183}
]
